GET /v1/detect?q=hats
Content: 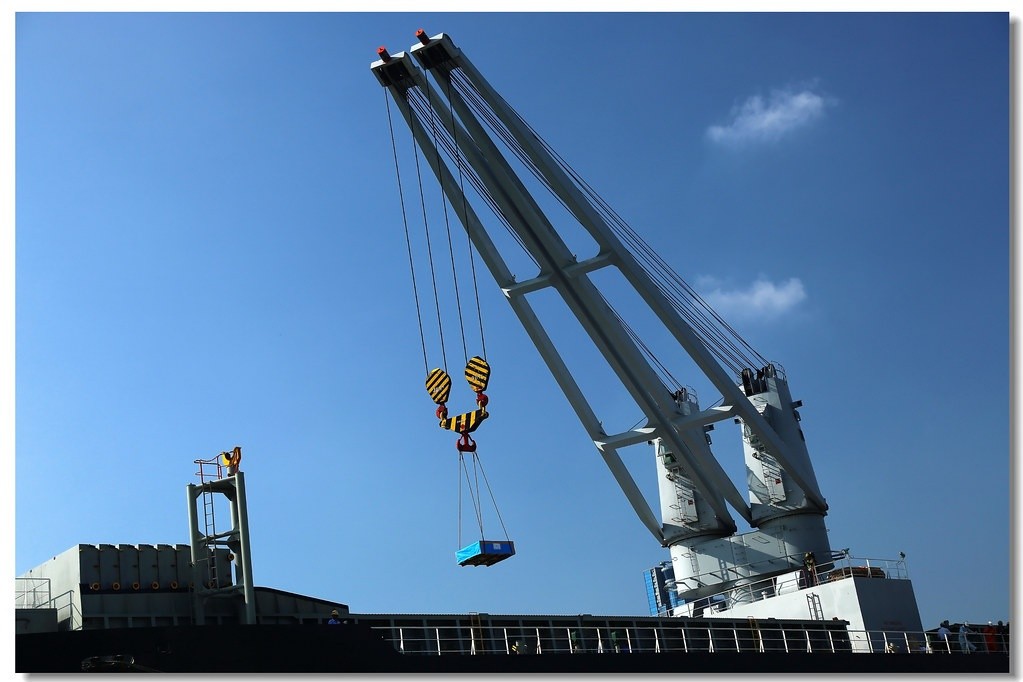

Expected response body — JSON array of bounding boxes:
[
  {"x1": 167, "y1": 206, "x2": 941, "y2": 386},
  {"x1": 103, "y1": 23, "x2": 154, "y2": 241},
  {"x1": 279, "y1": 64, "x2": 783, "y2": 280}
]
[{"x1": 965, "y1": 622, "x2": 969, "y2": 627}]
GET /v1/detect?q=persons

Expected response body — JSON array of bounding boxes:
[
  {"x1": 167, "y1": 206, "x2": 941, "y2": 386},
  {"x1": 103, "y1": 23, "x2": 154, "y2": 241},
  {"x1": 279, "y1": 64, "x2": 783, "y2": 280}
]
[
  {"x1": 936, "y1": 618, "x2": 1009, "y2": 655},
  {"x1": 328, "y1": 610, "x2": 341, "y2": 623}
]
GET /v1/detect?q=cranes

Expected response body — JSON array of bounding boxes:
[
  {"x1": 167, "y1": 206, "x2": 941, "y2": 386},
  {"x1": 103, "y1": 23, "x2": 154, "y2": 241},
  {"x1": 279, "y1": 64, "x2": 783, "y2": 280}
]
[{"x1": 363, "y1": 21, "x2": 925, "y2": 653}]
